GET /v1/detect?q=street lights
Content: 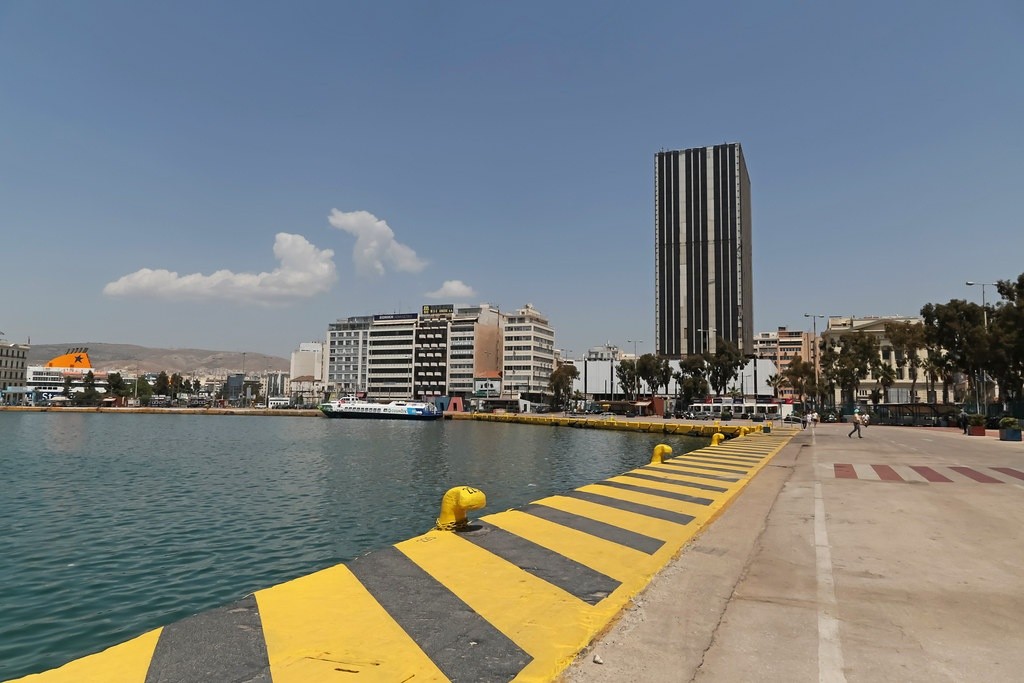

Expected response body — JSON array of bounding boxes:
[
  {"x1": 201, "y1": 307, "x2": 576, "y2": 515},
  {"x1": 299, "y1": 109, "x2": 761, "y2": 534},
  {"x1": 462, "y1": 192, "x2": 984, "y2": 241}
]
[
  {"x1": 628, "y1": 340, "x2": 643, "y2": 400},
  {"x1": 405, "y1": 355, "x2": 413, "y2": 399},
  {"x1": 965, "y1": 280, "x2": 998, "y2": 415},
  {"x1": 804, "y1": 313, "x2": 824, "y2": 405},
  {"x1": 698, "y1": 329, "x2": 717, "y2": 380},
  {"x1": 483, "y1": 351, "x2": 492, "y2": 398},
  {"x1": 561, "y1": 347, "x2": 573, "y2": 363}
]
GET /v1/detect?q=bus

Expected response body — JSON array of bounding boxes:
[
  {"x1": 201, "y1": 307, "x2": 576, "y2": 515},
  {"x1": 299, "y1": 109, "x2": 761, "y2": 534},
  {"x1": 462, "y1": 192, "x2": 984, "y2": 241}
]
[{"x1": 688, "y1": 403, "x2": 793, "y2": 418}]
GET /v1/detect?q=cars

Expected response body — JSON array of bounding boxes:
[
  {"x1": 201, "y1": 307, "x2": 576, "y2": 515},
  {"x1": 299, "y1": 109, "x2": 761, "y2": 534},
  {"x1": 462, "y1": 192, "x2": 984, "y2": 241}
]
[{"x1": 693, "y1": 411, "x2": 715, "y2": 420}]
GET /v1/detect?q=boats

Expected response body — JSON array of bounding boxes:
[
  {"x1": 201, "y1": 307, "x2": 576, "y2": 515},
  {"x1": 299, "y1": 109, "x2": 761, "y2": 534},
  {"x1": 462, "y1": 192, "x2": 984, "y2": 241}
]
[{"x1": 317, "y1": 394, "x2": 443, "y2": 421}]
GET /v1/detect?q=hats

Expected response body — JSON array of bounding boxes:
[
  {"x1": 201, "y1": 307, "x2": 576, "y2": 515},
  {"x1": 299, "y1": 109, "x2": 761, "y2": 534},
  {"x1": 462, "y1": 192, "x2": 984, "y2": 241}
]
[{"x1": 854, "y1": 409, "x2": 860, "y2": 413}]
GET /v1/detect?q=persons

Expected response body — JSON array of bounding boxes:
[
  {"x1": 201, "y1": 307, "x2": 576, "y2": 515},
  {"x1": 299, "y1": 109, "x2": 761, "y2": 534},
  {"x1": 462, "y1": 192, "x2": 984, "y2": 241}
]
[
  {"x1": 861, "y1": 413, "x2": 870, "y2": 421},
  {"x1": 963, "y1": 414, "x2": 968, "y2": 434},
  {"x1": 848, "y1": 410, "x2": 863, "y2": 439},
  {"x1": 800, "y1": 409, "x2": 819, "y2": 430}
]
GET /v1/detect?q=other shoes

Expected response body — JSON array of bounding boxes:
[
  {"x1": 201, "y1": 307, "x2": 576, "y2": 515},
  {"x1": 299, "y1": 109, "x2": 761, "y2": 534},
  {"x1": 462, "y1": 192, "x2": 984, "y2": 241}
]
[
  {"x1": 849, "y1": 435, "x2": 851, "y2": 438},
  {"x1": 859, "y1": 437, "x2": 863, "y2": 438}
]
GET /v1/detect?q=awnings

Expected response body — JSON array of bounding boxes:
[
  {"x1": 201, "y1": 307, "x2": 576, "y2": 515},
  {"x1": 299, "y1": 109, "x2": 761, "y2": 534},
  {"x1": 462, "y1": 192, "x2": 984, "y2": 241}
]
[{"x1": 635, "y1": 402, "x2": 651, "y2": 407}]
